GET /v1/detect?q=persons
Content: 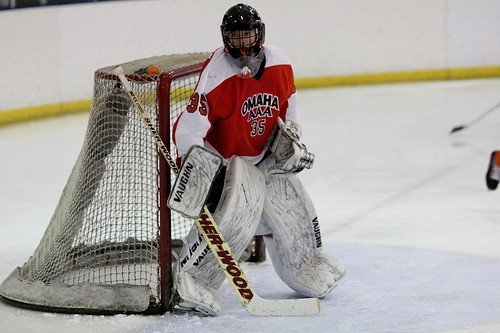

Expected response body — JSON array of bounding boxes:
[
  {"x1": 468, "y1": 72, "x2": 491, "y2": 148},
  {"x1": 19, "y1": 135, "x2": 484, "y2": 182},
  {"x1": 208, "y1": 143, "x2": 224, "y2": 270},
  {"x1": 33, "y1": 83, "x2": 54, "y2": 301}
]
[
  {"x1": 167, "y1": 4, "x2": 346, "y2": 316},
  {"x1": 486, "y1": 150, "x2": 500, "y2": 190}
]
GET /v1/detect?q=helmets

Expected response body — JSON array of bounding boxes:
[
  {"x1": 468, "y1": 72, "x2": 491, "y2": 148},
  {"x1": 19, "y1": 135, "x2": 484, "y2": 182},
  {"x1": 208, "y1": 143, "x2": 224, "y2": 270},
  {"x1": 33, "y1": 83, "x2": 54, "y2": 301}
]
[{"x1": 221, "y1": 3, "x2": 266, "y2": 59}]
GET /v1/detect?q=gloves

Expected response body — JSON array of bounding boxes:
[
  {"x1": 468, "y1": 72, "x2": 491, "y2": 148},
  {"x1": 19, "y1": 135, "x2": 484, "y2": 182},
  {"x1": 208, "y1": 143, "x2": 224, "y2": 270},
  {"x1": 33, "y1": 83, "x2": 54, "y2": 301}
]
[{"x1": 265, "y1": 120, "x2": 315, "y2": 179}]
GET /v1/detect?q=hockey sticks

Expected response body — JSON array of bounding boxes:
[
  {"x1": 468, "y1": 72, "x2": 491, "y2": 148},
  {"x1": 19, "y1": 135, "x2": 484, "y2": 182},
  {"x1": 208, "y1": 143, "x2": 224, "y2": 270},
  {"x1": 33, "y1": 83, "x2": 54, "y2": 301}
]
[
  {"x1": 450, "y1": 102, "x2": 500, "y2": 134},
  {"x1": 112, "y1": 64, "x2": 322, "y2": 318}
]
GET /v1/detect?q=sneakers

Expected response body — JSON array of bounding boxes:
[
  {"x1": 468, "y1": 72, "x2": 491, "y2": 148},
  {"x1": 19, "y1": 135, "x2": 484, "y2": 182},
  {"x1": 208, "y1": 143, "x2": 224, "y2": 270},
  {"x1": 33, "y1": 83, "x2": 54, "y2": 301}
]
[{"x1": 486, "y1": 150, "x2": 500, "y2": 190}]
